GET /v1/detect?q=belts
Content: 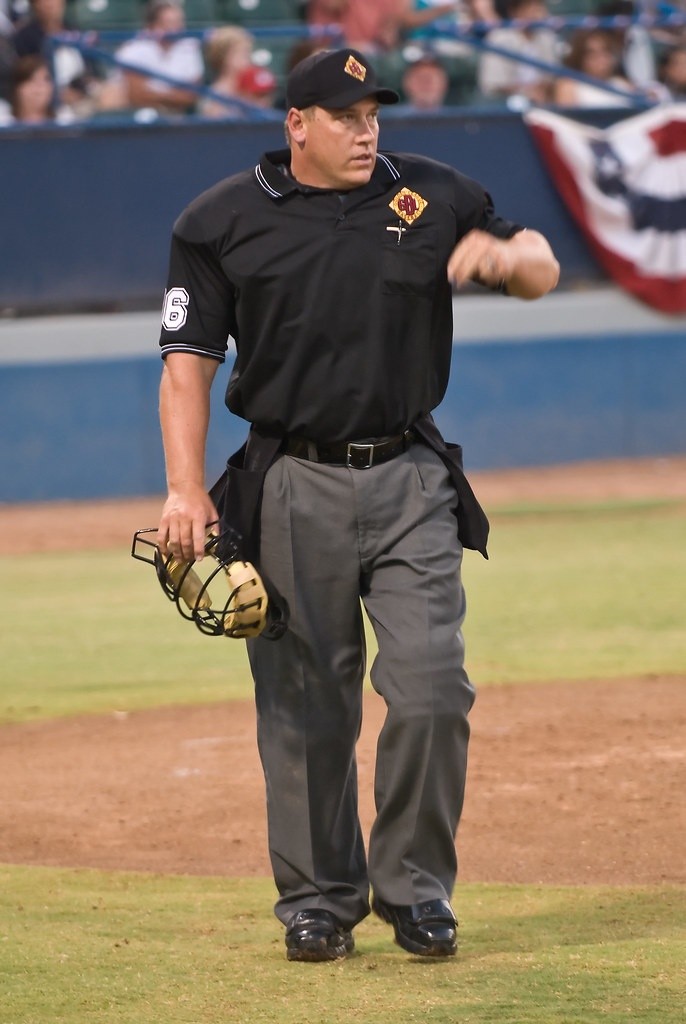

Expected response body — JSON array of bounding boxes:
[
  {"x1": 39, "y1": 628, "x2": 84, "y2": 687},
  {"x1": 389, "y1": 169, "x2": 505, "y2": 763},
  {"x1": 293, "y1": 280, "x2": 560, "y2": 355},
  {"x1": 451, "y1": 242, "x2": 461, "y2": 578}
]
[{"x1": 276, "y1": 430, "x2": 417, "y2": 470}]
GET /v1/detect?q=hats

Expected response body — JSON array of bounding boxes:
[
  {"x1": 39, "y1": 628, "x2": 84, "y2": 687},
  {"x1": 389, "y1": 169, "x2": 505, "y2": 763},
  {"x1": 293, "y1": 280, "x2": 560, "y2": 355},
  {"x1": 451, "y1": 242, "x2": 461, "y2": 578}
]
[{"x1": 286, "y1": 49, "x2": 400, "y2": 111}]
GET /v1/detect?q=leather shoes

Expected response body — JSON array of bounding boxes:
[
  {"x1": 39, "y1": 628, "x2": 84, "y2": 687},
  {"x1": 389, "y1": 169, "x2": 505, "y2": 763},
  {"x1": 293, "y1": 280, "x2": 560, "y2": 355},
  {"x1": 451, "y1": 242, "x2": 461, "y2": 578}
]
[
  {"x1": 285, "y1": 909, "x2": 354, "y2": 962},
  {"x1": 371, "y1": 892, "x2": 458, "y2": 957}
]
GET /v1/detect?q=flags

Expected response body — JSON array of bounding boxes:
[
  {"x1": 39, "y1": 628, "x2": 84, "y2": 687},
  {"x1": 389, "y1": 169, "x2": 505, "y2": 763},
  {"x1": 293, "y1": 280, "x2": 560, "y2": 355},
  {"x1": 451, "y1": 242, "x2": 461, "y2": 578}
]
[{"x1": 519, "y1": 105, "x2": 686, "y2": 317}]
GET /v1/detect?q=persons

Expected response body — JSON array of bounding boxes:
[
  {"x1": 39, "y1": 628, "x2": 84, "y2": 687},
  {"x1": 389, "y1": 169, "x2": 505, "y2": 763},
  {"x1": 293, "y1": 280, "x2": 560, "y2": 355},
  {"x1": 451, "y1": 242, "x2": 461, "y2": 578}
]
[
  {"x1": 0, "y1": 0, "x2": 686, "y2": 111},
  {"x1": 157, "y1": 48, "x2": 559, "y2": 963}
]
